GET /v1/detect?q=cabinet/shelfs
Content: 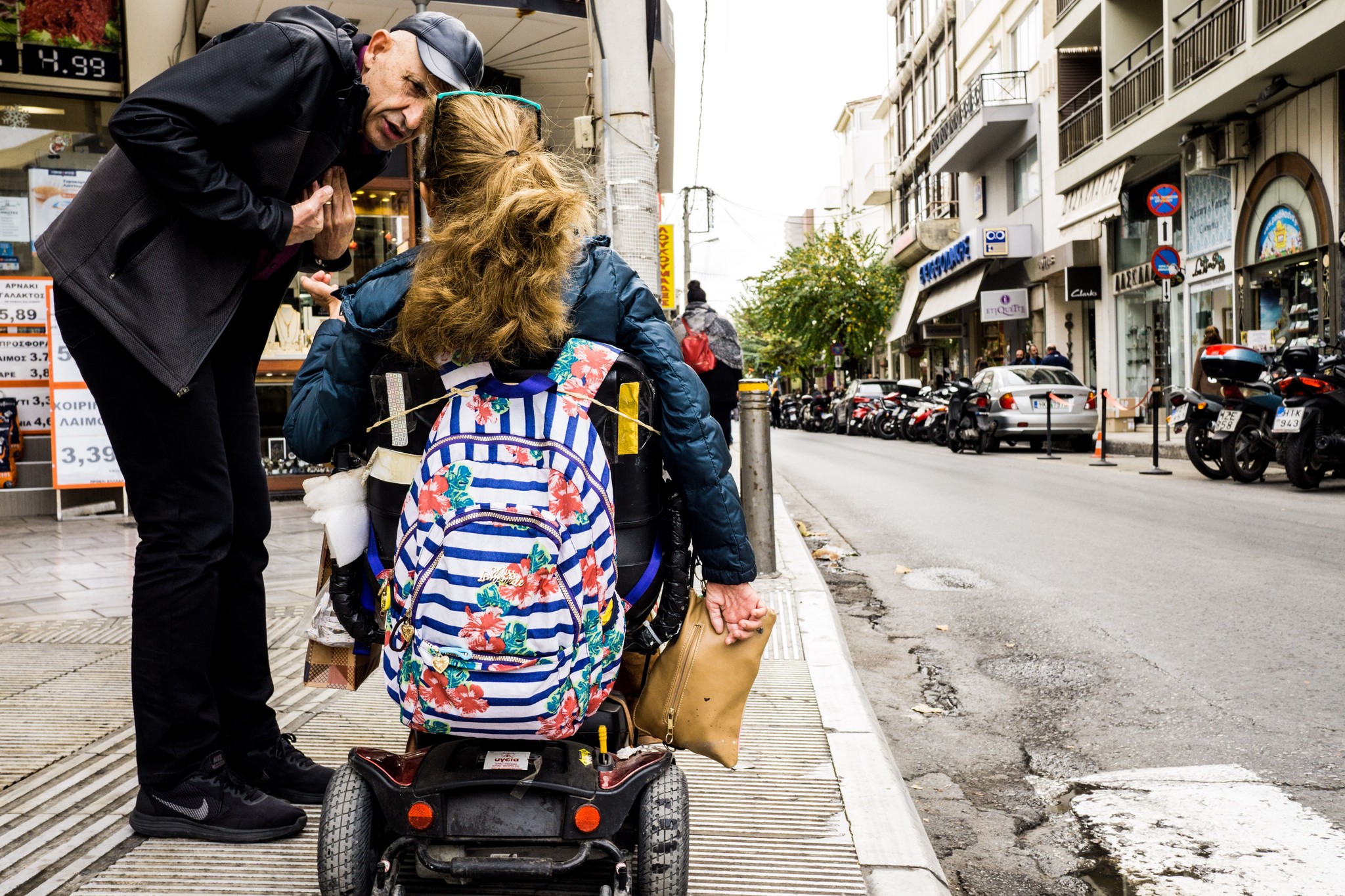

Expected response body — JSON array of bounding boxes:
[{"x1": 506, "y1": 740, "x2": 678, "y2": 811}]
[{"x1": 254, "y1": 358, "x2": 331, "y2": 500}]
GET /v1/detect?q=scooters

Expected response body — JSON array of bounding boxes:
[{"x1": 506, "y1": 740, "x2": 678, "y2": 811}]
[
  {"x1": 765, "y1": 390, "x2": 841, "y2": 432},
  {"x1": 1162, "y1": 337, "x2": 1345, "y2": 488},
  {"x1": 850, "y1": 367, "x2": 954, "y2": 448},
  {"x1": 943, "y1": 378, "x2": 996, "y2": 455},
  {"x1": 731, "y1": 408, "x2": 739, "y2": 422}
]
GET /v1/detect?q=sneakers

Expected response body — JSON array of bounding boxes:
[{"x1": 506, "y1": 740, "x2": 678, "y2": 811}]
[
  {"x1": 130, "y1": 749, "x2": 307, "y2": 843},
  {"x1": 221, "y1": 732, "x2": 336, "y2": 804}
]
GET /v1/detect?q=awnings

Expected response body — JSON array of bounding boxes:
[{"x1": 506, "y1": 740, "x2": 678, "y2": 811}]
[{"x1": 916, "y1": 264, "x2": 997, "y2": 326}]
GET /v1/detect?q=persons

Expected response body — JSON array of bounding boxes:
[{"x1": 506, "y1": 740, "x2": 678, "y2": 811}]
[
  {"x1": 1021, "y1": 345, "x2": 1041, "y2": 365},
  {"x1": 1010, "y1": 351, "x2": 1021, "y2": 364},
  {"x1": 1041, "y1": 344, "x2": 1071, "y2": 371},
  {"x1": 769, "y1": 391, "x2": 782, "y2": 428},
  {"x1": 284, "y1": 91, "x2": 768, "y2": 649},
  {"x1": 935, "y1": 374, "x2": 946, "y2": 388},
  {"x1": 1192, "y1": 327, "x2": 1224, "y2": 399},
  {"x1": 32, "y1": 5, "x2": 485, "y2": 843},
  {"x1": 671, "y1": 280, "x2": 743, "y2": 448}
]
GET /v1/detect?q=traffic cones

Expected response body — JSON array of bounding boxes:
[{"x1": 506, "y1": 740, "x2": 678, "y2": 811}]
[{"x1": 1092, "y1": 431, "x2": 1110, "y2": 458}]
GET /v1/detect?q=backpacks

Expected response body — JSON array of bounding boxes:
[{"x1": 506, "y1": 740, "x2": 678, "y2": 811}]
[
  {"x1": 681, "y1": 317, "x2": 716, "y2": 373},
  {"x1": 381, "y1": 335, "x2": 627, "y2": 740}
]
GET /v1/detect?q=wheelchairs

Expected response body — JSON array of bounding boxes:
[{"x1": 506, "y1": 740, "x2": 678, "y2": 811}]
[{"x1": 318, "y1": 344, "x2": 691, "y2": 896}]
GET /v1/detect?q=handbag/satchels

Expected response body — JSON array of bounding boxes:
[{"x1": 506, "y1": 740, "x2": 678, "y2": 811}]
[
  {"x1": 632, "y1": 589, "x2": 777, "y2": 769},
  {"x1": 294, "y1": 576, "x2": 359, "y2": 649},
  {"x1": 302, "y1": 524, "x2": 382, "y2": 692}
]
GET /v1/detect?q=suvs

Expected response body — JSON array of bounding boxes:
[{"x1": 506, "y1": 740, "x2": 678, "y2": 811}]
[{"x1": 833, "y1": 377, "x2": 899, "y2": 435}]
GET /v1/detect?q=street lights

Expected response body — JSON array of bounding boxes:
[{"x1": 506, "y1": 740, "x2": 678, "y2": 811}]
[{"x1": 685, "y1": 237, "x2": 717, "y2": 305}]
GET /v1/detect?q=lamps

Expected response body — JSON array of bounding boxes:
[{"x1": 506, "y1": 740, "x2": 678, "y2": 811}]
[{"x1": 1245, "y1": 102, "x2": 1257, "y2": 113}]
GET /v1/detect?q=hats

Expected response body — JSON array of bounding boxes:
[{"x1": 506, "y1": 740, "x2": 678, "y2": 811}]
[
  {"x1": 687, "y1": 280, "x2": 707, "y2": 303},
  {"x1": 390, "y1": 10, "x2": 484, "y2": 91}
]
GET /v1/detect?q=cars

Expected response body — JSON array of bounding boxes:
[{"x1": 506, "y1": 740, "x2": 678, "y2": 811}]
[{"x1": 971, "y1": 365, "x2": 1099, "y2": 449}]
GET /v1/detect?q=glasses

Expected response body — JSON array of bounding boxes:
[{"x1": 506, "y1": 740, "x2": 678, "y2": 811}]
[{"x1": 430, "y1": 93, "x2": 542, "y2": 163}]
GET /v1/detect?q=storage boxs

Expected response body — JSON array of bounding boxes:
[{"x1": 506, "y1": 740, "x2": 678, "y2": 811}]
[{"x1": 1098, "y1": 398, "x2": 1140, "y2": 432}]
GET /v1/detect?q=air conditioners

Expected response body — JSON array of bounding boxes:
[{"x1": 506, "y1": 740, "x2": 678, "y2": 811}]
[
  {"x1": 895, "y1": 35, "x2": 914, "y2": 68},
  {"x1": 1181, "y1": 121, "x2": 1249, "y2": 176}
]
